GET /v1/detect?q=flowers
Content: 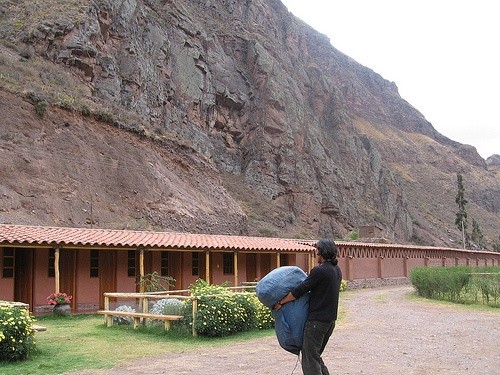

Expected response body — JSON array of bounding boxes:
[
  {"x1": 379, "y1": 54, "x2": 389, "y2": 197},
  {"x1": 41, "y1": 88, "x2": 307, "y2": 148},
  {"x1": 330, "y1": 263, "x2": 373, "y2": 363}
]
[{"x1": 47, "y1": 293, "x2": 72, "y2": 305}]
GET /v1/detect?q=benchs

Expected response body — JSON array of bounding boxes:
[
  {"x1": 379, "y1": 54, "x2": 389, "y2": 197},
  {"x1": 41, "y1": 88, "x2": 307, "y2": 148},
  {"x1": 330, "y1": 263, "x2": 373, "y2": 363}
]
[{"x1": 98, "y1": 310, "x2": 185, "y2": 330}]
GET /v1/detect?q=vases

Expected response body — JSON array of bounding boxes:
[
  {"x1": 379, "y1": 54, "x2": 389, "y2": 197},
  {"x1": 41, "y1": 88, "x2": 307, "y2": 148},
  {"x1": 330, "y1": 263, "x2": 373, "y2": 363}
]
[{"x1": 53, "y1": 302, "x2": 71, "y2": 316}]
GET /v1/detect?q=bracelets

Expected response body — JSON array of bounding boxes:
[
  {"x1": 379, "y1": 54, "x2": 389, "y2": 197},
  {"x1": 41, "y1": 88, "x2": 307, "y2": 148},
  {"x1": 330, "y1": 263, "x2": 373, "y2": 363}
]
[{"x1": 278, "y1": 301, "x2": 283, "y2": 305}]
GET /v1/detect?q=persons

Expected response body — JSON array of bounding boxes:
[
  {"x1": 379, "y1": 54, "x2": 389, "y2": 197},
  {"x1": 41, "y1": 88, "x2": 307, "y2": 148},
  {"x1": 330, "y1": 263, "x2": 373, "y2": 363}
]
[{"x1": 274, "y1": 238, "x2": 342, "y2": 375}]
